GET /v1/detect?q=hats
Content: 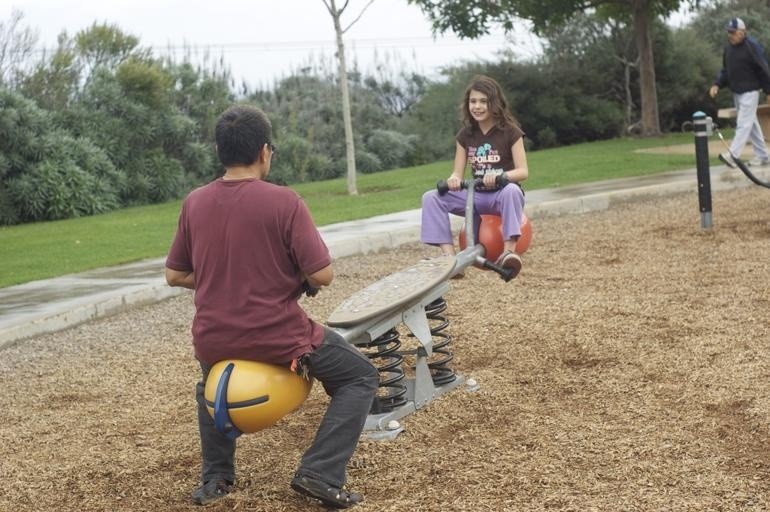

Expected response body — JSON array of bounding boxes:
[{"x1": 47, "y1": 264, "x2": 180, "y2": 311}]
[{"x1": 725, "y1": 17, "x2": 746, "y2": 32}]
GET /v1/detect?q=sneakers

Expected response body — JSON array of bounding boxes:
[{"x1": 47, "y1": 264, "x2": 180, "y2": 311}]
[
  {"x1": 718, "y1": 152, "x2": 738, "y2": 168},
  {"x1": 290, "y1": 473, "x2": 363, "y2": 508},
  {"x1": 189, "y1": 478, "x2": 231, "y2": 506},
  {"x1": 747, "y1": 157, "x2": 769, "y2": 166},
  {"x1": 422, "y1": 253, "x2": 464, "y2": 279},
  {"x1": 499, "y1": 249, "x2": 522, "y2": 280}
]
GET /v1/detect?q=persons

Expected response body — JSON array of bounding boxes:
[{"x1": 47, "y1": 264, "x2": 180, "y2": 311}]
[
  {"x1": 163, "y1": 105, "x2": 382, "y2": 508},
  {"x1": 420, "y1": 74, "x2": 531, "y2": 282},
  {"x1": 708, "y1": 17, "x2": 770, "y2": 169}
]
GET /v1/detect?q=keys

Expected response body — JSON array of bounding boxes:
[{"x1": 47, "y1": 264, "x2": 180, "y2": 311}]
[{"x1": 290, "y1": 354, "x2": 315, "y2": 382}]
